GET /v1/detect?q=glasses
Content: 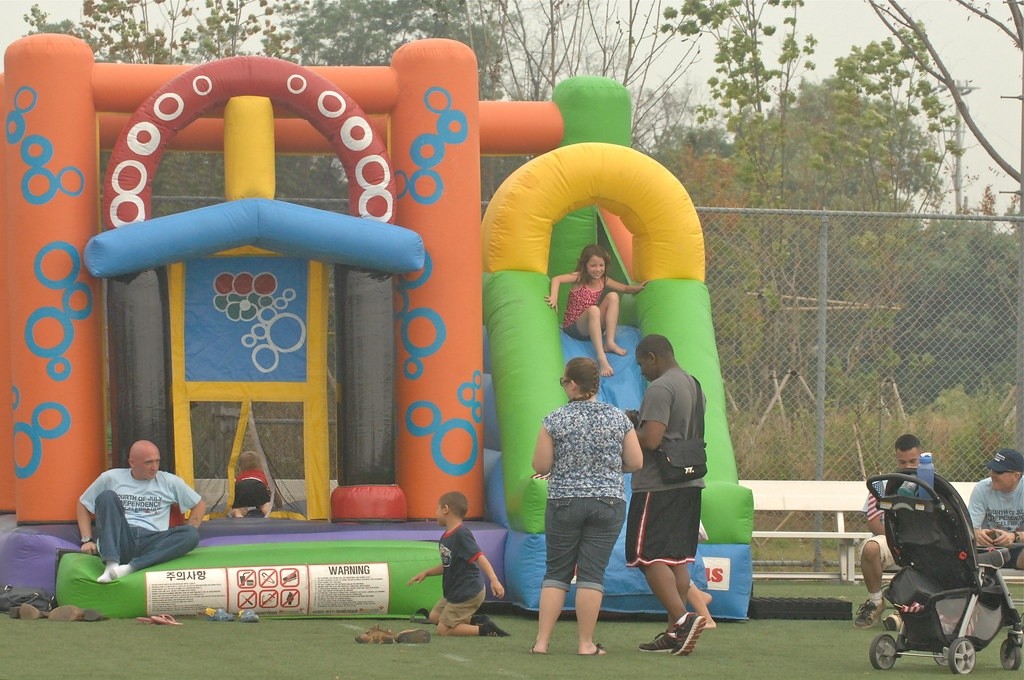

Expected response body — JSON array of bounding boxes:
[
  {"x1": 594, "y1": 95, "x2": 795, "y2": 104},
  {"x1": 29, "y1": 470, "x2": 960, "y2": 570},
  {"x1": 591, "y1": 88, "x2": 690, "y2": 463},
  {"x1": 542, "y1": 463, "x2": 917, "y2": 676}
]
[{"x1": 560, "y1": 378, "x2": 573, "y2": 386}]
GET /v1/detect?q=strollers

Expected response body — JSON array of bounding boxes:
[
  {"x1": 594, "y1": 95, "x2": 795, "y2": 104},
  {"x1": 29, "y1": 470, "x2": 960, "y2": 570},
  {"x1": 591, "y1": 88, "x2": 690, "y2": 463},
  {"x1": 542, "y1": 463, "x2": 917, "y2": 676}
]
[{"x1": 862, "y1": 467, "x2": 1024, "y2": 677}]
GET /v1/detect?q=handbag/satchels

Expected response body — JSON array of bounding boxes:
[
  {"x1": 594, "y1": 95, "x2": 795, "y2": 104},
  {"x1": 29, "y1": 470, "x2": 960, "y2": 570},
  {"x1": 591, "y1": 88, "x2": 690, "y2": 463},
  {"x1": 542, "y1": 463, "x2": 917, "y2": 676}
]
[
  {"x1": 655, "y1": 437, "x2": 707, "y2": 484},
  {"x1": 0, "y1": 583, "x2": 58, "y2": 611}
]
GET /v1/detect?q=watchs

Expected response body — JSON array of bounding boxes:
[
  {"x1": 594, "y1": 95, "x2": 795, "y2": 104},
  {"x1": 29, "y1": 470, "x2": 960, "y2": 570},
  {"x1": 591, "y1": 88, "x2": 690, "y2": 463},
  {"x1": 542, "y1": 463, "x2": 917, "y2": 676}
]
[
  {"x1": 1012, "y1": 531, "x2": 1020, "y2": 543},
  {"x1": 80, "y1": 537, "x2": 94, "y2": 546}
]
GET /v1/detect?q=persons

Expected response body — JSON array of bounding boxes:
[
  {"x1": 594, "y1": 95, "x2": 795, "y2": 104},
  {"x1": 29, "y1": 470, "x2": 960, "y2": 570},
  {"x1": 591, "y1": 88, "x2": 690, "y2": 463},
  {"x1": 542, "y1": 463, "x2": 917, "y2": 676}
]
[
  {"x1": 544, "y1": 244, "x2": 650, "y2": 378},
  {"x1": 626, "y1": 334, "x2": 706, "y2": 656},
  {"x1": 407, "y1": 492, "x2": 511, "y2": 636},
  {"x1": 77, "y1": 440, "x2": 207, "y2": 583},
  {"x1": 968, "y1": 448, "x2": 1024, "y2": 633},
  {"x1": 854, "y1": 434, "x2": 923, "y2": 630},
  {"x1": 232, "y1": 451, "x2": 282, "y2": 518},
  {"x1": 532, "y1": 356, "x2": 643, "y2": 656}
]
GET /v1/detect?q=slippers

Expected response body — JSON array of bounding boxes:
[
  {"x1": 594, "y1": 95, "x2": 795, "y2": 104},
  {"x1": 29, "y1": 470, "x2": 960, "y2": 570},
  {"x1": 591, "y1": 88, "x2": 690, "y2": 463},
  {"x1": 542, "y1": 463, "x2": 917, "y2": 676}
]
[
  {"x1": 137, "y1": 617, "x2": 153, "y2": 624},
  {"x1": 578, "y1": 643, "x2": 604, "y2": 656},
  {"x1": 532, "y1": 644, "x2": 549, "y2": 653},
  {"x1": 410, "y1": 607, "x2": 432, "y2": 624},
  {"x1": 151, "y1": 614, "x2": 183, "y2": 626}
]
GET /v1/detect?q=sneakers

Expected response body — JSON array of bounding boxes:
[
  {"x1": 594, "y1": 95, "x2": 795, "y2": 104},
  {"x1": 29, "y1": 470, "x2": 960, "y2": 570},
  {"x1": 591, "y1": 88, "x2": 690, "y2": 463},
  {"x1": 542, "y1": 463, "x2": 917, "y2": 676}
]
[
  {"x1": 881, "y1": 612, "x2": 904, "y2": 630},
  {"x1": 354, "y1": 623, "x2": 393, "y2": 645},
  {"x1": 853, "y1": 597, "x2": 885, "y2": 630},
  {"x1": 396, "y1": 628, "x2": 431, "y2": 643},
  {"x1": 638, "y1": 629, "x2": 675, "y2": 653},
  {"x1": 671, "y1": 612, "x2": 706, "y2": 656}
]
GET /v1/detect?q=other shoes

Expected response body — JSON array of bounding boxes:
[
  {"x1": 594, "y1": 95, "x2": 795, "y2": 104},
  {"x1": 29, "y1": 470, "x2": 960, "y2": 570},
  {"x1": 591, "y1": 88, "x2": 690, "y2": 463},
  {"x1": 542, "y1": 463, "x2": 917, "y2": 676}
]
[
  {"x1": 8, "y1": 605, "x2": 47, "y2": 619},
  {"x1": 48, "y1": 605, "x2": 106, "y2": 621},
  {"x1": 483, "y1": 621, "x2": 510, "y2": 637},
  {"x1": 474, "y1": 615, "x2": 489, "y2": 625}
]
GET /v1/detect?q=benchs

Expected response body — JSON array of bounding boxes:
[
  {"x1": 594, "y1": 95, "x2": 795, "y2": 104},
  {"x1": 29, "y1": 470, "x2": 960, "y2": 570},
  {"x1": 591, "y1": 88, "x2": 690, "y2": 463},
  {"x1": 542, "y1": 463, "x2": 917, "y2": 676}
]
[{"x1": 738, "y1": 478, "x2": 1023, "y2": 585}]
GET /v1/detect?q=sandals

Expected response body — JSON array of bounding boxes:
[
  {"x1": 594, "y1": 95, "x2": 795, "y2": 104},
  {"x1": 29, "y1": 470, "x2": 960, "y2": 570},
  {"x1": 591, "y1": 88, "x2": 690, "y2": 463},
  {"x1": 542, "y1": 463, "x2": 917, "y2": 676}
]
[
  {"x1": 238, "y1": 609, "x2": 258, "y2": 622},
  {"x1": 205, "y1": 607, "x2": 235, "y2": 621}
]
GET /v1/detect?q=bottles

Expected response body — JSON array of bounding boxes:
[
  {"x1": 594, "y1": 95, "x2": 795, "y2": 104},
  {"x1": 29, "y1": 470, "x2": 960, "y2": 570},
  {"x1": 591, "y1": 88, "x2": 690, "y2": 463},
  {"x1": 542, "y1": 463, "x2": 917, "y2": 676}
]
[{"x1": 896, "y1": 452, "x2": 935, "y2": 500}]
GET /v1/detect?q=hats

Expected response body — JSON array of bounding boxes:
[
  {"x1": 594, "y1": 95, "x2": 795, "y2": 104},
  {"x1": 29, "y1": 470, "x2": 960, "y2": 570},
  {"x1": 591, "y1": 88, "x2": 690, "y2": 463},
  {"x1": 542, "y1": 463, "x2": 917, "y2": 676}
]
[{"x1": 986, "y1": 448, "x2": 1023, "y2": 474}]
